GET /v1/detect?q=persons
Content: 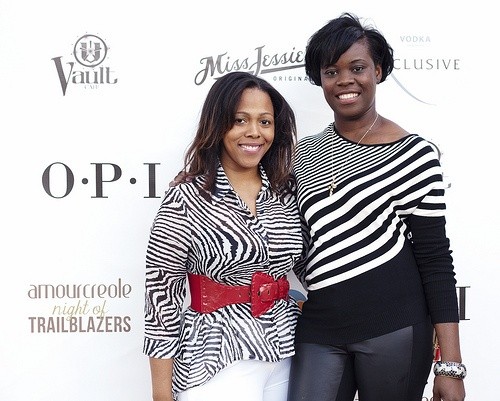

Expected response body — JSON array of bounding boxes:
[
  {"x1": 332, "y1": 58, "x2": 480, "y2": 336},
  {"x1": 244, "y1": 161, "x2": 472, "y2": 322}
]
[
  {"x1": 165, "y1": 13, "x2": 467, "y2": 401},
  {"x1": 143, "y1": 72, "x2": 303, "y2": 401}
]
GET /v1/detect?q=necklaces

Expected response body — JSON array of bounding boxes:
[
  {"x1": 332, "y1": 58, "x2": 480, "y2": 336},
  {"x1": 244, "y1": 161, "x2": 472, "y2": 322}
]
[{"x1": 329, "y1": 111, "x2": 378, "y2": 196}]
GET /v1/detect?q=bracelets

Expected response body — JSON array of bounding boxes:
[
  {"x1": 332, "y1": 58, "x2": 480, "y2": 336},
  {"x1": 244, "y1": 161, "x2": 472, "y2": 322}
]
[{"x1": 433, "y1": 361, "x2": 467, "y2": 380}]
